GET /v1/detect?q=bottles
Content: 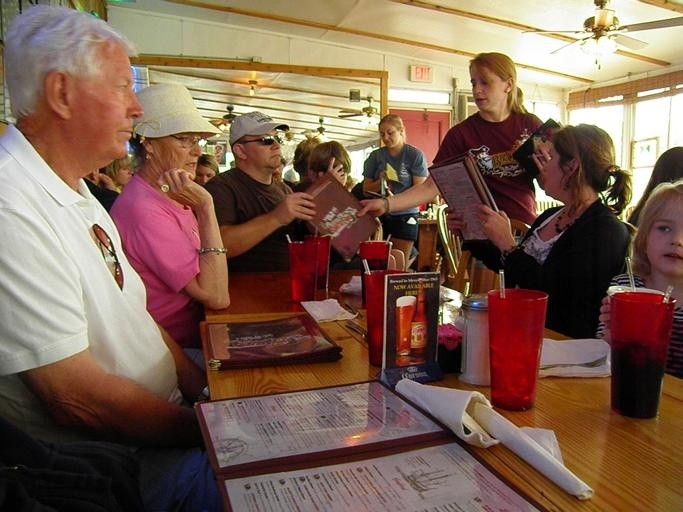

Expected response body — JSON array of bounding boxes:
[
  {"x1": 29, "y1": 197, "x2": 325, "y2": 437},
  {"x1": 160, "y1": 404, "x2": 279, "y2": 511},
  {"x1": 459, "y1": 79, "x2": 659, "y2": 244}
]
[
  {"x1": 396, "y1": 297, "x2": 416, "y2": 367},
  {"x1": 459, "y1": 296, "x2": 490, "y2": 387},
  {"x1": 411, "y1": 280, "x2": 428, "y2": 362}
]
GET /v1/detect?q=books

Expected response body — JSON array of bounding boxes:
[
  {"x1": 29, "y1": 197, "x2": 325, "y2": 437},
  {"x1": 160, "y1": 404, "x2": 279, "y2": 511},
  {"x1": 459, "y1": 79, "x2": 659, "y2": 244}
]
[
  {"x1": 302, "y1": 173, "x2": 380, "y2": 262},
  {"x1": 511, "y1": 118, "x2": 565, "y2": 178},
  {"x1": 364, "y1": 177, "x2": 403, "y2": 200},
  {"x1": 426, "y1": 154, "x2": 501, "y2": 242},
  {"x1": 201, "y1": 314, "x2": 344, "y2": 370}
]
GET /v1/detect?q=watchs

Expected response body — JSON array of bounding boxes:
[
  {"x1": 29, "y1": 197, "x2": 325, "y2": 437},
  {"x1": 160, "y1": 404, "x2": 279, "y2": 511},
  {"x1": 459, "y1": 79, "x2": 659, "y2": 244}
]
[{"x1": 193, "y1": 385, "x2": 210, "y2": 404}]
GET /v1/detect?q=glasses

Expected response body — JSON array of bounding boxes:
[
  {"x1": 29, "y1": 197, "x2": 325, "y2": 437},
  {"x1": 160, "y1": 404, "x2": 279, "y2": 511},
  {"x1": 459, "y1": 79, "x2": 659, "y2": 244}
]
[
  {"x1": 92, "y1": 220, "x2": 124, "y2": 293},
  {"x1": 233, "y1": 134, "x2": 284, "y2": 146},
  {"x1": 168, "y1": 136, "x2": 210, "y2": 147}
]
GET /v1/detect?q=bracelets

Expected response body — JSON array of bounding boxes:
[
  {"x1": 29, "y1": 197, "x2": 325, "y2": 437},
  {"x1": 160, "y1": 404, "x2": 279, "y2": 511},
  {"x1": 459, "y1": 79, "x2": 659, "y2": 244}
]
[
  {"x1": 199, "y1": 247, "x2": 228, "y2": 254},
  {"x1": 385, "y1": 196, "x2": 391, "y2": 213}
]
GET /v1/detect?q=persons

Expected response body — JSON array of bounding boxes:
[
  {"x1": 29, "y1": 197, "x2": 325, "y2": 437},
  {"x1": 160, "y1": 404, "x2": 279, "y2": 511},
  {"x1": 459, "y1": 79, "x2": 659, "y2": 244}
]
[
  {"x1": 443, "y1": 124, "x2": 631, "y2": 338},
  {"x1": 356, "y1": 52, "x2": 553, "y2": 296},
  {"x1": 597, "y1": 180, "x2": 683, "y2": 378},
  {"x1": 202, "y1": 111, "x2": 316, "y2": 273},
  {"x1": 109, "y1": 84, "x2": 229, "y2": 369},
  {"x1": 291, "y1": 135, "x2": 322, "y2": 191},
  {"x1": 1, "y1": 5, "x2": 223, "y2": 510},
  {"x1": 105, "y1": 156, "x2": 134, "y2": 193},
  {"x1": 300, "y1": 141, "x2": 372, "y2": 269},
  {"x1": 363, "y1": 115, "x2": 429, "y2": 270},
  {"x1": 83, "y1": 168, "x2": 119, "y2": 211},
  {"x1": 194, "y1": 154, "x2": 218, "y2": 187},
  {"x1": 628, "y1": 145, "x2": 683, "y2": 229}
]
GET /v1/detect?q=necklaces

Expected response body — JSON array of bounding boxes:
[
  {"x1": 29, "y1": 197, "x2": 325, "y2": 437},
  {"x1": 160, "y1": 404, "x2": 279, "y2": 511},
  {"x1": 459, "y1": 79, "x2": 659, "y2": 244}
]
[{"x1": 556, "y1": 207, "x2": 573, "y2": 232}]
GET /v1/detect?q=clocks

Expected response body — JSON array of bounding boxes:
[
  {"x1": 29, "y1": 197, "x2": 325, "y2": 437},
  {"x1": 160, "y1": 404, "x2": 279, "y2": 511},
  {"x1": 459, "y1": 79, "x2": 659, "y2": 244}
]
[{"x1": 409, "y1": 64, "x2": 433, "y2": 83}]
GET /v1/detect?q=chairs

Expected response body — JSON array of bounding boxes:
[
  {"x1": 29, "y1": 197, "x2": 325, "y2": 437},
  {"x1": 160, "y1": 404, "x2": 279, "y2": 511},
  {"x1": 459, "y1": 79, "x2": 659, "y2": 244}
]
[{"x1": 436, "y1": 202, "x2": 471, "y2": 298}]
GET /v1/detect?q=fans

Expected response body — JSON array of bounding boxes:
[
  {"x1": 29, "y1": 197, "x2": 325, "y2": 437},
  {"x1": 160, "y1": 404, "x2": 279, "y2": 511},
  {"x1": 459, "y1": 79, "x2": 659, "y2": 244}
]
[
  {"x1": 301, "y1": 125, "x2": 343, "y2": 142},
  {"x1": 337, "y1": 106, "x2": 380, "y2": 118},
  {"x1": 520, "y1": 0, "x2": 682, "y2": 54},
  {"x1": 201, "y1": 104, "x2": 240, "y2": 129}
]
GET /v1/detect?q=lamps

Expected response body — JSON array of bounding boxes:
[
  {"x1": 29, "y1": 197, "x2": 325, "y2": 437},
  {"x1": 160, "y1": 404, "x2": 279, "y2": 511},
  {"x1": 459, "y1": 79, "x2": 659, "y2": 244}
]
[
  {"x1": 248, "y1": 88, "x2": 255, "y2": 96},
  {"x1": 577, "y1": 35, "x2": 618, "y2": 72}
]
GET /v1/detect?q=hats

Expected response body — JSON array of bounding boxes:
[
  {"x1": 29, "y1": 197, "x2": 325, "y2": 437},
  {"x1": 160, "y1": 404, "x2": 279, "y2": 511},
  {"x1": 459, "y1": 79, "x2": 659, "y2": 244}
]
[
  {"x1": 127, "y1": 82, "x2": 223, "y2": 142},
  {"x1": 228, "y1": 111, "x2": 289, "y2": 144}
]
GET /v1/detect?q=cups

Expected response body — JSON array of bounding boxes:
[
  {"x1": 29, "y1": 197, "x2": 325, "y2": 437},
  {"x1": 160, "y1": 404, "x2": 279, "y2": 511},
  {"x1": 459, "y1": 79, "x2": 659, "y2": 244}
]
[
  {"x1": 305, "y1": 234, "x2": 331, "y2": 290},
  {"x1": 609, "y1": 291, "x2": 676, "y2": 417},
  {"x1": 364, "y1": 269, "x2": 407, "y2": 367},
  {"x1": 488, "y1": 288, "x2": 547, "y2": 411},
  {"x1": 396, "y1": 297, "x2": 416, "y2": 356},
  {"x1": 360, "y1": 240, "x2": 391, "y2": 304},
  {"x1": 609, "y1": 286, "x2": 665, "y2": 300},
  {"x1": 288, "y1": 241, "x2": 319, "y2": 304}
]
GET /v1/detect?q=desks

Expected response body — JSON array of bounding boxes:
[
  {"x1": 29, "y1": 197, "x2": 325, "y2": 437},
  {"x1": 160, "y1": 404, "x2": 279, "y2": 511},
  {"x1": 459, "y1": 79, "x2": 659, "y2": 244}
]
[{"x1": 199, "y1": 267, "x2": 683, "y2": 512}]
